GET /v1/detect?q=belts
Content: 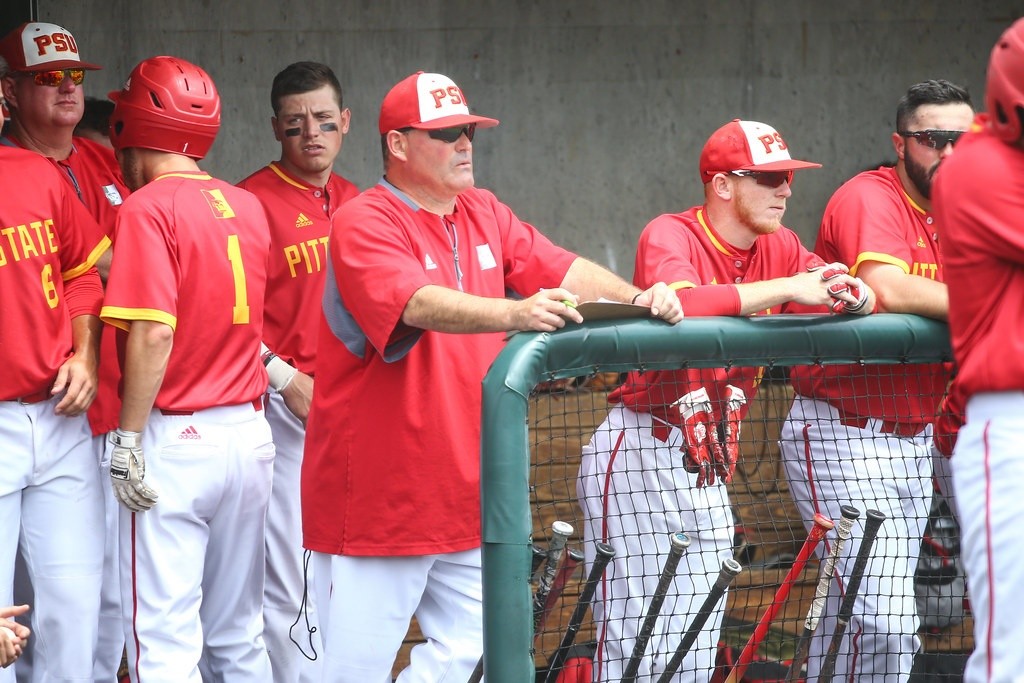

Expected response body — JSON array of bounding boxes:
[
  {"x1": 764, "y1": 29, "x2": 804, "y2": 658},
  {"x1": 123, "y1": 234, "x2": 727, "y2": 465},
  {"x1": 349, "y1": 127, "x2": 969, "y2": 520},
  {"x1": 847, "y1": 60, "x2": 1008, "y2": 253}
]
[
  {"x1": 160, "y1": 391, "x2": 263, "y2": 416},
  {"x1": 14, "y1": 382, "x2": 70, "y2": 403},
  {"x1": 838, "y1": 408, "x2": 931, "y2": 436},
  {"x1": 650, "y1": 418, "x2": 687, "y2": 453}
]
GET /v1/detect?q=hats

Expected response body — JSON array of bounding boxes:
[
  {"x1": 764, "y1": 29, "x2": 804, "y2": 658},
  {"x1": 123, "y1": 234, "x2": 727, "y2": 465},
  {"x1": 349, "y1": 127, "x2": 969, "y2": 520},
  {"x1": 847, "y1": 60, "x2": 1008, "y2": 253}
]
[
  {"x1": 0, "y1": 22, "x2": 103, "y2": 78},
  {"x1": 699, "y1": 119, "x2": 822, "y2": 184},
  {"x1": 379, "y1": 71, "x2": 499, "y2": 134}
]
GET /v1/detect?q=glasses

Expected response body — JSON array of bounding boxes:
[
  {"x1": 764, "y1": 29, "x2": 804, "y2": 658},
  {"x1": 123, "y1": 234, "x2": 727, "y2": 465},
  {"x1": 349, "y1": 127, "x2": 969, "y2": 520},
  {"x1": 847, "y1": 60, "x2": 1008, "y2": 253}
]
[
  {"x1": 397, "y1": 121, "x2": 475, "y2": 143},
  {"x1": 4, "y1": 67, "x2": 85, "y2": 86},
  {"x1": 707, "y1": 170, "x2": 794, "y2": 188},
  {"x1": 898, "y1": 130, "x2": 967, "y2": 151}
]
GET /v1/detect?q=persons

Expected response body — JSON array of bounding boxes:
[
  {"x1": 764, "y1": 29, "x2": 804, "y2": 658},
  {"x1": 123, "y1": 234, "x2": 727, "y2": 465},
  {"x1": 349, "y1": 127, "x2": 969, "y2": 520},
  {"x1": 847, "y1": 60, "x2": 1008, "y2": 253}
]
[
  {"x1": 99, "y1": 55, "x2": 277, "y2": 683},
  {"x1": 301, "y1": 70, "x2": 682, "y2": 683},
  {"x1": 237, "y1": 60, "x2": 362, "y2": 682},
  {"x1": 580, "y1": 117, "x2": 876, "y2": 683},
  {"x1": 781, "y1": 79, "x2": 976, "y2": 683},
  {"x1": 0, "y1": 18, "x2": 136, "y2": 683},
  {"x1": 932, "y1": 18, "x2": 1024, "y2": 683},
  {"x1": 870, "y1": 162, "x2": 973, "y2": 630}
]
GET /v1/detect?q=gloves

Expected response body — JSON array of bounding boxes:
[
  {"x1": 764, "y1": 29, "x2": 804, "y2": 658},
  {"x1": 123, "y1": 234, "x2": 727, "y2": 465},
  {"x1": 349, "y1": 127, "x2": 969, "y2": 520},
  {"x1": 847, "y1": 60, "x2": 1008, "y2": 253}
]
[
  {"x1": 673, "y1": 386, "x2": 731, "y2": 489},
  {"x1": 108, "y1": 428, "x2": 157, "y2": 512},
  {"x1": 806, "y1": 260, "x2": 868, "y2": 313},
  {"x1": 718, "y1": 383, "x2": 746, "y2": 484}
]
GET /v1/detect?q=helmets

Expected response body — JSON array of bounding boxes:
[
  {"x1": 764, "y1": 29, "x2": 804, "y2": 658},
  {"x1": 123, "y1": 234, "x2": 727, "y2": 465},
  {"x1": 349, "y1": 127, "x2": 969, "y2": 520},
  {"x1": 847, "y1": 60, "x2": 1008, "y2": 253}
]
[
  {"x1": 106, "y1": 55, "x2": 221, "y2": 162},
  {"x1": 985, "y1": 16, "x2": 1024, "y2": 143}
]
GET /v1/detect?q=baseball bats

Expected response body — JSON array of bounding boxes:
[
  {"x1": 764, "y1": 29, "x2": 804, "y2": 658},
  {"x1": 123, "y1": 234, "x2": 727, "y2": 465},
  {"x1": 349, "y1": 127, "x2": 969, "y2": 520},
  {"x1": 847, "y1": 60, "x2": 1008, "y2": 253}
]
[
  {"x1": 536, "y1": 547, "x2": 586, "y2": 643},
  {"x1": 657, "y1": 557, "x2": 743, "y2": 683},
  {"x1": 816, "y1": 509, "x2": 887, "y2": 682},
  {"x1": 532, "y1": 520, "x2": 575, "y2": 633},
  {"x1": 784, "y1": 504, "x2": 860, "y2": 682},
  {"x1": 545, "y1": 542, "x2": 618, "y2": 683},
  {"x1": 466, "y1": 546, "x2": 548, "y2": 683},
  {"x1": 621, "y1": 531, "x2": 691, "y2": 683},
  {"x1": 727, "y1": 512, "x2": 836, "y2": 683}
]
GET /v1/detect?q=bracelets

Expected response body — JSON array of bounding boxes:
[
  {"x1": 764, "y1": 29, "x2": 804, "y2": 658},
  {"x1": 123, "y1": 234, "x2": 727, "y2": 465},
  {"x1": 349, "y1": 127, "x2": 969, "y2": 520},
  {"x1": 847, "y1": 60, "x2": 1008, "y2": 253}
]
[{"x1": 632, "y1": 292, "x2": 642, "y2": 304}]
